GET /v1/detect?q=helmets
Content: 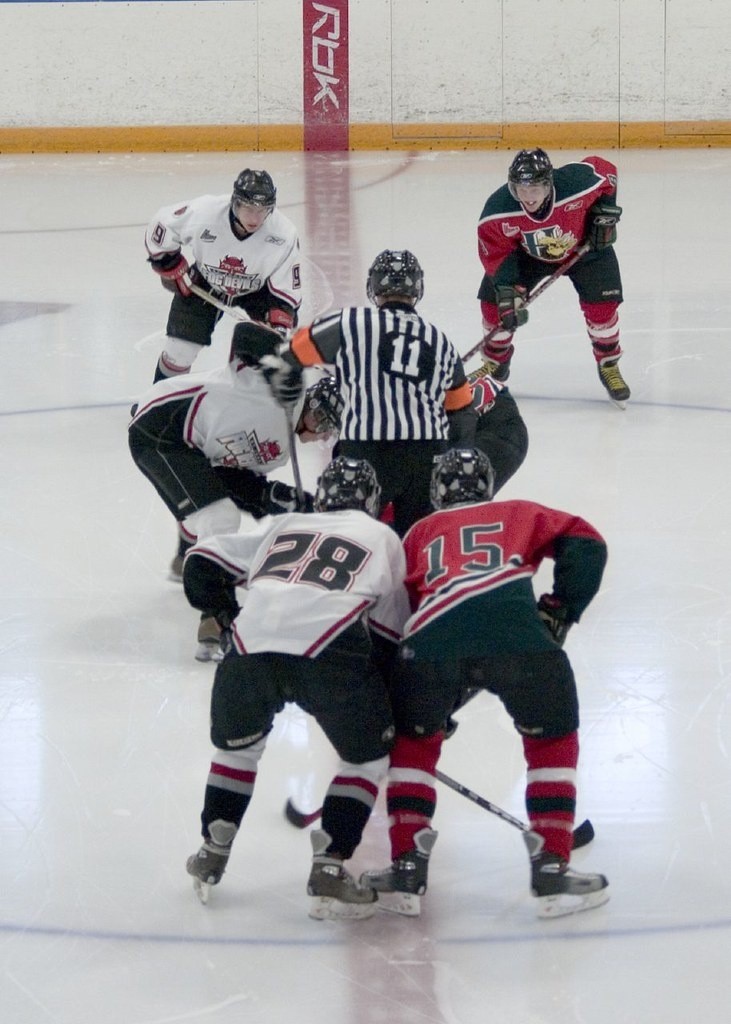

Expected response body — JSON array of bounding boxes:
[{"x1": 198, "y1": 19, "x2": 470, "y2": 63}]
[
  {"x1": 303, "y1": 375, "x2": 343, "y2": 431},
  {"x1": 367, "y1": 249, "x2": 422, "y2": 302},
  {"x1": 310, "y1": 456, "x2": 380, "y2": 517},
  {"x1": 233, "y1": 168, "x2": 276, "y2": 215},
  {"x1": 428, "y1": 447, "x2": 494, "y2": 511},
  {"x1": 508, "y1": 147, "x2": 553, "y2": 195}
]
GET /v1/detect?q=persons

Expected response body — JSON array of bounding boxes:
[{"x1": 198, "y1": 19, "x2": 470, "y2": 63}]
[
  {"x1": 145, "y1": 168, "x2": 301, "y2": 385},
  {"x1": 129, "y1": 322, "x2": 343, "y2": 664},
  {"x1": 181, "y1": 455, "x2": 409, "y2": 922},
  {"x1": 474, "y1": 149, "x2": 630, "y2": 408},
  {"x1": 417, "y1": 374, "x2": 529, "y2": 501},
  {"x1": 287, "y1": 249, "x2": 513, "y2": 536},
  {"x1": 360, "y1": 447, "x2": 609, "y2": 920}
]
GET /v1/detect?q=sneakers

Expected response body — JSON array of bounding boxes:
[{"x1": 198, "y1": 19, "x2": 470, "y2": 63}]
[
  {"x1": 195, "y1": 615, "x2": 225, "y2": 661},
  {"x1": 360, "y1": 827, "x2": 439, "y2": 915},
  {"x1": 598, "y1": 352, "x2": 631, "y2": 410},
  {"x1": 524, "y1": 830, "x2": 608, "y2": 918},
  {"x1": 305, "y1": 829, "x2": 378, "y2": 920},
  {"x1": 465, "y1": 354, "x2": 510, "y2": 385},
  {"x1": 186, "y1": 819, "x2": 237, "y2": 903}
]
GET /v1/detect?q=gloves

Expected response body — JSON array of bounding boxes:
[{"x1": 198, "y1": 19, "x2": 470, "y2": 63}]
[
  {"x1": 266, "y1": 304, "x2": 296, "y2": 336},
  {"x1": 265, "y1": 364, "x2": 302, "y2": 409},
  {"x1": 539, "y1": 590, "x2": 574, "y2": 647},
  {"x1": 588, "y1": 202, "x2": 623, "y2": 251},
  {"x1": 263, "y1": 479, "x2": 312, "y2": 514},
  {"x1": 149, "y1": 250, "x2": 194, "y2": 296},
  {"x1": 494, "y1": 283, "x2": 529, "y2": 328}
]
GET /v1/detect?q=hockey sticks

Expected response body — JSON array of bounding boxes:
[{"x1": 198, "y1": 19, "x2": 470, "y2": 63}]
[
  {"x1": 459, "y1": 242, "x2": 594, "y2": 363},
  {"x1": 284, "y1": 799, "x2": 323, "y2": 832},
  {"x1": 283, "y1": 402, "x2": 307, "y2": 505},
  {"x1": 435, "y1": 769, "x2": 595, "y2": 851},
  {"x1": 191, "y1": 284, "x2": 285, "y2": 336}
]
